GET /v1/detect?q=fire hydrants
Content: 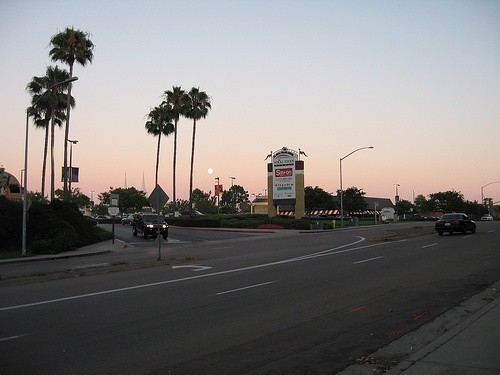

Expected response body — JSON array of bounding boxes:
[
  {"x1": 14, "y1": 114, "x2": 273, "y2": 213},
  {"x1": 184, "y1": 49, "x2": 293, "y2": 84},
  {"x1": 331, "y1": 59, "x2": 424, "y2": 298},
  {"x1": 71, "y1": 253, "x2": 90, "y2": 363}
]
[{"x1": 332, "y1": 220, "x2": 335, "y2": 229}]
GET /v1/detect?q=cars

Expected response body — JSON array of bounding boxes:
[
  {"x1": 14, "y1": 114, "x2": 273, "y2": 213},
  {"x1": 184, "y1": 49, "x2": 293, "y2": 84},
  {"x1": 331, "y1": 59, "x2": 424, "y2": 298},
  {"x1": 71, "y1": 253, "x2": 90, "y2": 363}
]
[
  {"x1": 121, "y1": 216, "x2": 131, "y2": 225},
  {"x1": 131, "y1": 212, "x2": 169, "y2": 240},
  {"x1": 91, "y1": 215, "x2": 110, "y2": 223},
  {"x1": 481, "y1": 214, "x2": 493, "y2": 222},
  {"x1": 164, "y1": 210, "x2": 203, "y2": 217},
  {"x1": 435, "y1": 213, "x2": 477, "y2": 235}
]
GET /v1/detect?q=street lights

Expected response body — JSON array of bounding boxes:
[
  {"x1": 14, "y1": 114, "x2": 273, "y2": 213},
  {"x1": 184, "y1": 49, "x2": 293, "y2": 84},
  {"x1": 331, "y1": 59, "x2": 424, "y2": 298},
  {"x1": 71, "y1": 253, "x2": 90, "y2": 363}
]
[
  {"x1": 68, "y1": 139, "x2": 78, "y2": 194},
  {"x1": 215, "y1": 177, "x2": 220, "y2": 207},
  {"x1": 252, "y1": 193, "x2": 262, "y2": 197},
  {"x1": 392, "y1": 183, "x2": 400, "y2": 223},
  {"x1": 21, "y1": 76, "x2": 79, "y2": 256},
  {"x1": 229, "y1": 177, "x2": 235, "y2": 186},
  {"x1": 339, "y1": 146, "x2": 375, "y2": 228},
  {"x1": 91, "y1": 190, "x2": 95, "y2": 217}
]
